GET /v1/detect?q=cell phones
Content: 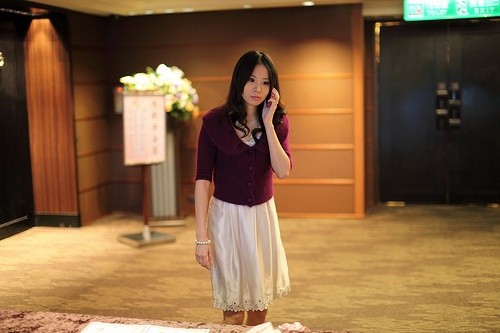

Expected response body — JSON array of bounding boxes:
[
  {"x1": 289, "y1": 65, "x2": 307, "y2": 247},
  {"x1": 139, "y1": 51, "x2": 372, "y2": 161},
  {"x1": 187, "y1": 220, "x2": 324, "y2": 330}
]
[{"x1": 266, "y1": 93, "x2": 274, "y2": 107}]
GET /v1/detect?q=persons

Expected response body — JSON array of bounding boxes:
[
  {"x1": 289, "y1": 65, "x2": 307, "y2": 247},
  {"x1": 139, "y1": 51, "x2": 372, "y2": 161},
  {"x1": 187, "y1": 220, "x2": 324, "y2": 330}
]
[{"x1": 195, "y1": 49, "x2": 293, "y2": 327}]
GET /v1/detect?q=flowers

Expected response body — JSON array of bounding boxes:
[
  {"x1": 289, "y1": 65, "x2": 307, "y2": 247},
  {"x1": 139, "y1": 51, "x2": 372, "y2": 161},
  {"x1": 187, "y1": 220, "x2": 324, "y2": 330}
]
[{"x1": 120, "y1": 63, "x2": 201, "y2": 121}]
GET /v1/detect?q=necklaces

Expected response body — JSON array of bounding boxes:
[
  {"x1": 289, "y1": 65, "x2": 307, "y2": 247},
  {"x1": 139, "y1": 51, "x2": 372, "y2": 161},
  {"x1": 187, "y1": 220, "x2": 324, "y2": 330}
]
[{"x1": 236, "y1": 120, "x2": 261, "y2": 139}]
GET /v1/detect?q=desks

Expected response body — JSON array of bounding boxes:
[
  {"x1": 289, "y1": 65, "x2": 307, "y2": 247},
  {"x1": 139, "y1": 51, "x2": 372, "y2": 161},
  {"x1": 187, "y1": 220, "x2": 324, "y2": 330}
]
[{"x1": 0, "y1": 309, "x2": 347, "y2": 333}]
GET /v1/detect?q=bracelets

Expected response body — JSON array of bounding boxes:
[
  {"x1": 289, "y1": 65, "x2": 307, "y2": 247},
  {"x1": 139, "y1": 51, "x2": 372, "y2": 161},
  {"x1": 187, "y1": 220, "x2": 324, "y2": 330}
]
[{"x1": 195, "y1": 239, "x2": 211, "y2": 245}]
